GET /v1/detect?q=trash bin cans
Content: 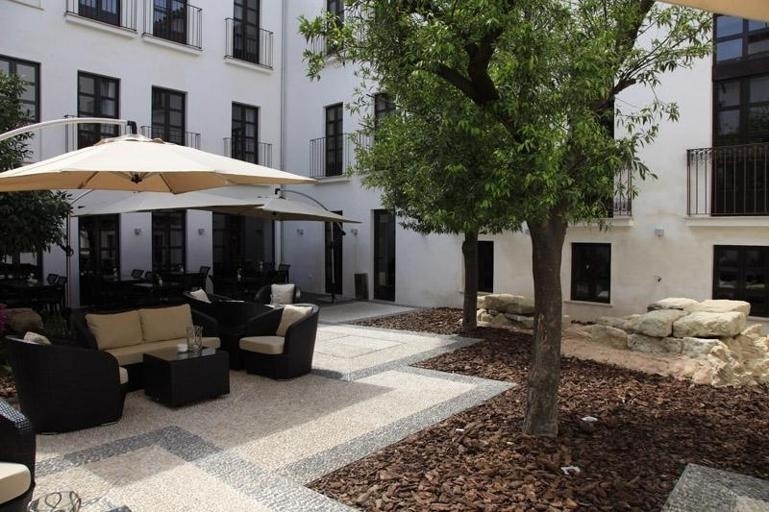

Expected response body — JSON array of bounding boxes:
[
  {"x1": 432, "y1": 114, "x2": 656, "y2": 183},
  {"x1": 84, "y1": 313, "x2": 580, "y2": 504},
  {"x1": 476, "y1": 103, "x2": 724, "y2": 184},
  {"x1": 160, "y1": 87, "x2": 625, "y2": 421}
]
[{"x1": 355, "y1": 273, "x2": 368, "y2": 300}]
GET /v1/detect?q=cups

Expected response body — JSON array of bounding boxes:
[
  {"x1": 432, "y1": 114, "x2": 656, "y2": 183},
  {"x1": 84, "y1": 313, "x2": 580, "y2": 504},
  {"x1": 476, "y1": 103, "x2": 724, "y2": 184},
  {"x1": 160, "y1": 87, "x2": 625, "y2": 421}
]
[
  {"x1": 177, "y1": 343, "x2": 187, "y2": 352},
  {"x1": 185, "y1": 325, "x2": 203, "y2": 353}
]
